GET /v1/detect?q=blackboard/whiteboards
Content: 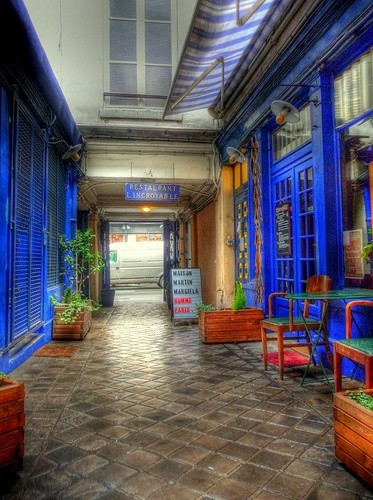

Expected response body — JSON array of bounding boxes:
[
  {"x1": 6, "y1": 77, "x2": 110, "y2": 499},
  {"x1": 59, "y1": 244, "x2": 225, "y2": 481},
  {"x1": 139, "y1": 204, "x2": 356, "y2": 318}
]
[{"x1": 169, "y1": 267, "x2": 203, "y2": 321}]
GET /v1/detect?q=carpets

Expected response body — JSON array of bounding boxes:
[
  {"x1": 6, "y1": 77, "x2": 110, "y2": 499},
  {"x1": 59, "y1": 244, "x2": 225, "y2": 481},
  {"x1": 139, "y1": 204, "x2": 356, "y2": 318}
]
[{"x1": 32, "y1": 344, "x2": 79, "y2": 357}]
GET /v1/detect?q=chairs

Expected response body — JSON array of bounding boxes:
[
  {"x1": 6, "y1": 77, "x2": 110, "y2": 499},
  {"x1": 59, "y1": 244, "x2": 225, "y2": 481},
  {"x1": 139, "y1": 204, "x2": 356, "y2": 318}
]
[
  {"x1": 332, "y1": 300, "x2": 372, "y2": 393},
  {"x1": 259, "y1": 273, "x2": 336, "y2": 381}
]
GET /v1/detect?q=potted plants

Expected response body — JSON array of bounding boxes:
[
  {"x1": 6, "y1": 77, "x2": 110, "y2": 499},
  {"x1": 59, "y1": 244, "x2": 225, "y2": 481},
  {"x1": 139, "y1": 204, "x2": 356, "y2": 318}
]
[
  {"x1": 100, "y1": 284, "x2": 116, "y2": 306},
  {"x1": 194, "y1": 280, "x2": 265, "y2": 344},
  {"x1": 49, "y1": 225, "x2": 109, "y2": 341},
  {"x1": 333, "y1": 387, "x2": 373, "y2": 495}
]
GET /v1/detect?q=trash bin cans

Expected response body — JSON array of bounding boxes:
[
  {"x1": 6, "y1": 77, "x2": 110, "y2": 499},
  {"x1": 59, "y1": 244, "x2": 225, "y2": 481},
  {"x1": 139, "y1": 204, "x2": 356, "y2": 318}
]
[{"x1": 99, "y1": 289, "x2": 115, "y2": 306}]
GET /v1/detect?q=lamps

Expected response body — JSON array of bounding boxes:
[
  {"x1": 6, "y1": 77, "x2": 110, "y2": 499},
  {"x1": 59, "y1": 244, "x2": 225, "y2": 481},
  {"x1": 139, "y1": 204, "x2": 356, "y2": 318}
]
[
  {"x1": 225, "y1": 128, "x2": 259, "y2": 164},
  {"x1": 41, "y1": 106, "x2": 84, "y2": 162},
  {"x1": 270, "y1": 87, "x2": 322, "y2": 123}
]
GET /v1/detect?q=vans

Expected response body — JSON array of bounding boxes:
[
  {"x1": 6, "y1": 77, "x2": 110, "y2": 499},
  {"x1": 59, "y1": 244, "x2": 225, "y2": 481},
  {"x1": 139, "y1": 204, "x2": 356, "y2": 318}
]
[{"x1": 109, "y1": 241, "x2": 164, "y2": 289}]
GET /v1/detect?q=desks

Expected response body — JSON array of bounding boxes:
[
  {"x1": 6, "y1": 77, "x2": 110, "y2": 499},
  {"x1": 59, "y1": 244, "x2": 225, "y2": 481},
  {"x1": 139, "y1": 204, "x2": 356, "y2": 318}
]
[{"x1": 284, "y1": 287, "x2": 372, "y2": 396}]
